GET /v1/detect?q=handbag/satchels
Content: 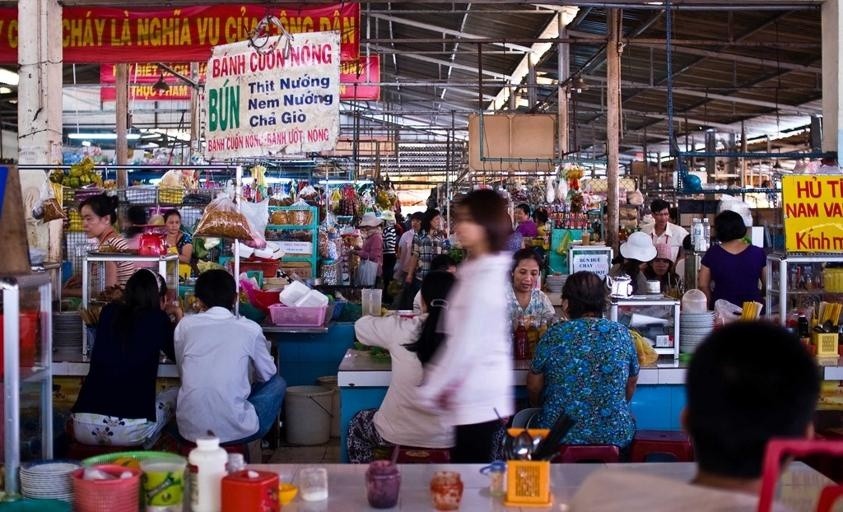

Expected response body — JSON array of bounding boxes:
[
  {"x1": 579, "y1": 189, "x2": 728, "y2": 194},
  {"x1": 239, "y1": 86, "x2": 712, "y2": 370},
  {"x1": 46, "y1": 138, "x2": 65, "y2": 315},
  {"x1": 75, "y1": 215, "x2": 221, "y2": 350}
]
[{"x1": 359, "y1": 253, "x2": 379, "y2": 286}]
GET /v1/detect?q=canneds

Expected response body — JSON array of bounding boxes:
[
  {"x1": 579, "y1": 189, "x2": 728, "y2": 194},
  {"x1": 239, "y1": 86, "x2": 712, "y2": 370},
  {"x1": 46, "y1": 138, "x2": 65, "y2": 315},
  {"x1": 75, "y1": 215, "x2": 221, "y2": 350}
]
[{"x1": 364, "y1": 461, "x2": 401, "y2": 509}]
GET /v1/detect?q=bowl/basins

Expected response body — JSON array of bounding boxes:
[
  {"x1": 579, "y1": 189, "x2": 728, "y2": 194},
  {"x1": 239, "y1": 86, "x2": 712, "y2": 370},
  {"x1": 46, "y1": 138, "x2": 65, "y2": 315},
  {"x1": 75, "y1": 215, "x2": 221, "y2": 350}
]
[{"x1": 274, "y1": 484, "x2": 296, "y2": 504}]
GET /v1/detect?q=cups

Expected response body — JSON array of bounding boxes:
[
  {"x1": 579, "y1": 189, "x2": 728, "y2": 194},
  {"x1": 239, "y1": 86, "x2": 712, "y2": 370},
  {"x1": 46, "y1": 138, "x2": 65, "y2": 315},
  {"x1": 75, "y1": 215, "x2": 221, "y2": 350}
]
[
  {"x1": 369, "y1": 463, "x2": 400, "y2": 508},
  {"x1": 647, "y1": 280, "x2": 659, "y2": 293},
  {"x1": 360, "y1": 289, "x2": 382, "y2": 318},
  {"x1": 301, "y1": 470, "x2": 328, "y2": 500},
  {"x1": 142, "y1": 461, "x2": 184, "y2": 512}
]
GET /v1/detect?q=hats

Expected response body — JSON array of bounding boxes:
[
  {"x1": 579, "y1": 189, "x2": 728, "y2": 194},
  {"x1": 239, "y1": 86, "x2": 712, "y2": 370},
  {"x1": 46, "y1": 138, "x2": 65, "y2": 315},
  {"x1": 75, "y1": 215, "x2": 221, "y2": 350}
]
[
  {"x1": 620, "y1": 231, "x2": 658, "y2": 262},
  {"x1": 359, "y1": 211, "x2": 383, "y2": 228},
  {"x1": 380, "y1": 210, "x2": 395, "y2": 221},
  {"x1": 654, "y1": 244, "x2": 674, "y2": 262}
]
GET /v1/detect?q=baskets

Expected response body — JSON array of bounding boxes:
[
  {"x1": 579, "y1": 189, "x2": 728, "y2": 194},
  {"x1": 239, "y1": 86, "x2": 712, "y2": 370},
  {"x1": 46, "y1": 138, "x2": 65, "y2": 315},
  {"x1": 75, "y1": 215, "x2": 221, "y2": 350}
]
[{"x1": 267, "y1": 304, "x2": 329, "y2": 326}]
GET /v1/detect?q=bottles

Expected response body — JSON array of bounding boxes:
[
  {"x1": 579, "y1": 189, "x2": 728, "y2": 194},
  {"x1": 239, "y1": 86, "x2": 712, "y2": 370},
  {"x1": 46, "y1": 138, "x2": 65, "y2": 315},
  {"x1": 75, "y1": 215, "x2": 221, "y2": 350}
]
[
  {"x1": 512, "y1": 313, "x2": 549, "y2": 359},
  {"x1": 189, "y1": 437, "x2": 226, "y2": 511},
  {"x1": 690, "y1": 218, "x2": 709, "y2": 251},
  {"x1": 797, "y1": 311, "x2": 809, "y2": 345}
]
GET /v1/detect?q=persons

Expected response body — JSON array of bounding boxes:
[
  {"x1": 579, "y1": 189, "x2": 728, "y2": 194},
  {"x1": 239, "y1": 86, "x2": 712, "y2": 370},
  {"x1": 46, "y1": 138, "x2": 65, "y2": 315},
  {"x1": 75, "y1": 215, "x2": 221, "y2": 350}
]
[
  {"x1": 159, "y1": 209, "x2": 194, "y2": 264},
  {"x1": 116, "y1": 204, "x2": 144, "y2": 237},
  {"x1": 353, "y1": 207, "x2": 458, "y2": 315},
  {"x1": 345, "y1": 269, "x2": 458, "y2": 464},
  {"x1": 408, "y1": 188, "x2": 519, "y2": 463},
  {"x1": 506, "y1": 196, "x2": 695, "y2": 334},
  {"x1": 67, "y1": 267, "x2": 185, "y2": 451},
  {"x1": 697, "y1": 210, "x2": 769, "y2": 316},
  {"x1": 172, "y1": 268, "x2": 288, "y2": 449},
  {"x1": 57, "y1": 193, "x2": 136, "y2": 298},
  {"x1": 564, "y1": 316, "x2": 823, "y2": 511},
  {"x1": 525, "y1": 270, "x2": 641, "y2": 464}
]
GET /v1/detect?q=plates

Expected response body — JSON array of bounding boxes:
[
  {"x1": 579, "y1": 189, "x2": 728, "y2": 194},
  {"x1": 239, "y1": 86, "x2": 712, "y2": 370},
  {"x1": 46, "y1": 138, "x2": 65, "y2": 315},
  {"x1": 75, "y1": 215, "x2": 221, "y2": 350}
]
[
  {"x1": 681, "y1": 311, "x2": 715, "y2": 351},
  {"x1": 16, "y1": 461, "x2": 82, "y2": 512}
]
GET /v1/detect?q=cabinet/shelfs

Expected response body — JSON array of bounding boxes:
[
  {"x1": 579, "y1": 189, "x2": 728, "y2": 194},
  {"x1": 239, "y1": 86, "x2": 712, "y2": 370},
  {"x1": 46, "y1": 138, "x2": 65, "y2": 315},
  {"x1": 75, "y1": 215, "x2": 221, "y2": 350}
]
[
  {"x1": 264, "y1": 202, "x2": 321, "y2": 284},
  {"x1": 77, "y1": 250, "x2": 178, "y2": 361},
  {"x1": 604, "y1": 290, "x2": 680, "y2": 365},
  {"x1": 765, "y1": 251, "x2": 842, "y2": 334}
]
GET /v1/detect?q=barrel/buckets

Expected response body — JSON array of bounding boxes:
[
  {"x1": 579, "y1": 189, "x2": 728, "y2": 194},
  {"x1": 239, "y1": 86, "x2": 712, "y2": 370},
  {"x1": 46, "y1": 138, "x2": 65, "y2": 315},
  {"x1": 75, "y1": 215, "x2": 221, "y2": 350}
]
[
  {"x1": 318, "y1": 376, "x2": 340, "y2": 441},
  {"x1": 284, "y1": 384, "x2": 332, "y2": 446}
]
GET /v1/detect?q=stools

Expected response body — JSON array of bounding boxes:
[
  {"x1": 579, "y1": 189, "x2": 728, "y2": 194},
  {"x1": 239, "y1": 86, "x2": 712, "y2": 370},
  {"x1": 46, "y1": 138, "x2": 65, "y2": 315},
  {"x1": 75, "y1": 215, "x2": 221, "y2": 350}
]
[
  {"x1": 558, "y1": 426, "x2": 695, "y2": 462},
  {"x1": 66, "y1": 435, "x2": 249, "y2": 471}
]
